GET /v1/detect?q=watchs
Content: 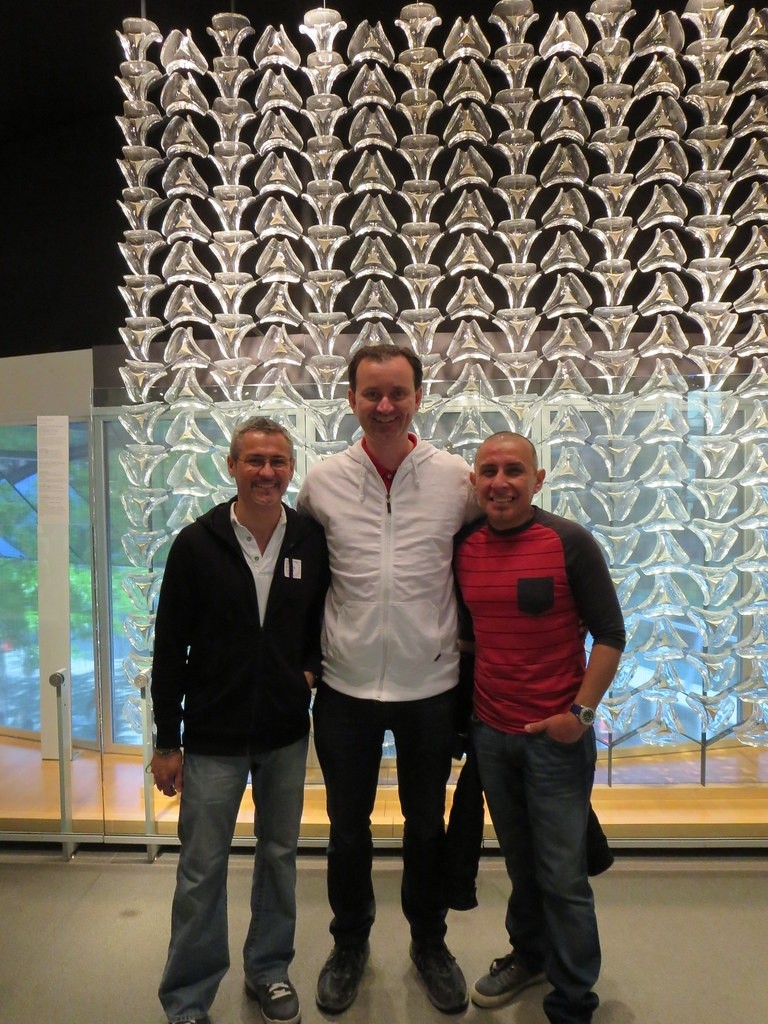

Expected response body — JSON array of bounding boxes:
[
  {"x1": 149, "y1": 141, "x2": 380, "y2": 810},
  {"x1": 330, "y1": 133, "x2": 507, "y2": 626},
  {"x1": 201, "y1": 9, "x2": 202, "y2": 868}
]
[{"x1": 569, "y1": 703, "x2": 596, "y2": 726}]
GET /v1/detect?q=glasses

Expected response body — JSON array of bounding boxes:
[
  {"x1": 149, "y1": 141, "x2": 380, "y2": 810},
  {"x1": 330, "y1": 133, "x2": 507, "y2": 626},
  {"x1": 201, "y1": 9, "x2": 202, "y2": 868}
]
[{"x1": 236, "y1": 454, "x2": 292, "y2": 471}]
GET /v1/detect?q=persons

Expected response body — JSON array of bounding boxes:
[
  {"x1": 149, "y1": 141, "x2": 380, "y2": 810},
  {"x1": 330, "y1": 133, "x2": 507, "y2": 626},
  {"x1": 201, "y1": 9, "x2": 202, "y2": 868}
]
[
  {"x1": 151, "y1": 416, "x2": 331, "y2": 1024},
  {"x1": 294, "y1": 344, "x2": 487, "y2": 1015},
  {"x1": 449, "y1": 429, "x2": 627, "y2": 1024}
]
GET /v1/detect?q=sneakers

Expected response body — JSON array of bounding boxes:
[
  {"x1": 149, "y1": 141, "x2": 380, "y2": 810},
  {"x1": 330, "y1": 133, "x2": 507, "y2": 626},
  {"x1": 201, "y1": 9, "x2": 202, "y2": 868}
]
[
  {"x1": 169, "y1": 1014, "x2": 210, "y2": 1024},
  {"x1": 244, "y1": 979, "x2": 301, "y2": 1024},
  {"x1": 409, "y1": 940, "x2": 469, "y2": 1011},
  {"x1": 471, "y1": 950, "x2": 546, "y2": 1008},
  {"x1": 316, "y1": 940, "x2": 371, "y2": 1013}
]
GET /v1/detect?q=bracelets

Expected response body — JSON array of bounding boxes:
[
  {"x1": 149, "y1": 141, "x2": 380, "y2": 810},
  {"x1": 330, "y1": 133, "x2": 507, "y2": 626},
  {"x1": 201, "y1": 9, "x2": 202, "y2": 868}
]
[{"x1": 153, "y1": 748, "x2": 181, "y2": 757}]
